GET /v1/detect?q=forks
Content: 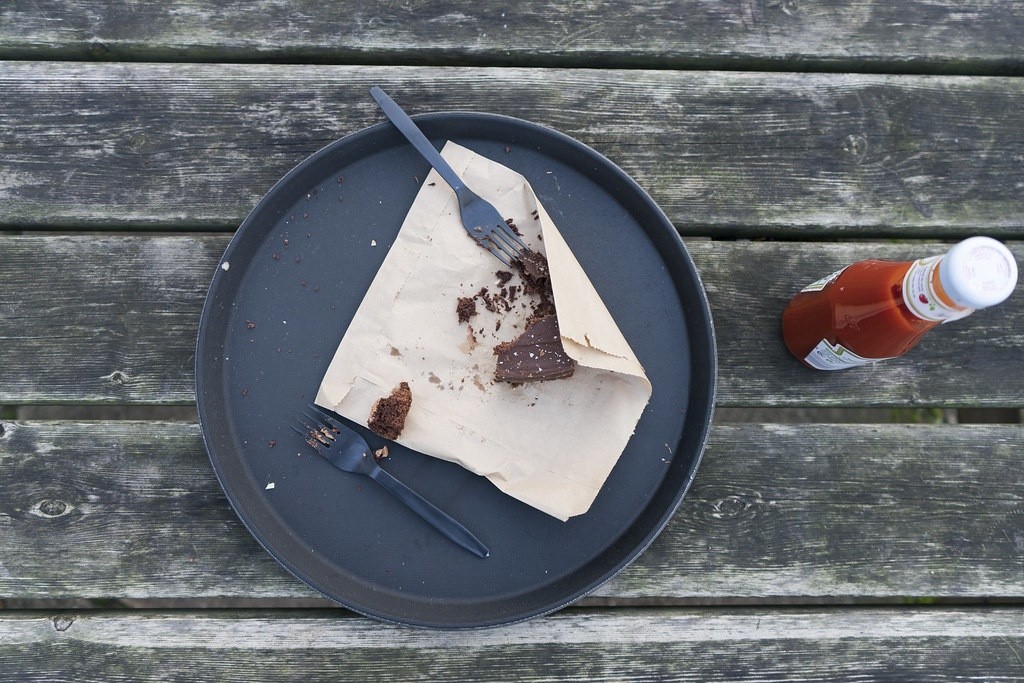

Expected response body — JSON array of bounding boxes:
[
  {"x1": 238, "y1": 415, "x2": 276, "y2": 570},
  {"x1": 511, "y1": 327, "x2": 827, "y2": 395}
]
[
  {"x1": 290, "y1": 402, "x2": 490, "y2": 558},
  {"x1": 369, "y1": 85, "x2": 530, "y2": 268}
]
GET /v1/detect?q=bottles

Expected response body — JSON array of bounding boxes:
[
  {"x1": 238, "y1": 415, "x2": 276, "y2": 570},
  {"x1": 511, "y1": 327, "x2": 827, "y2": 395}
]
[{"x1": 779, "y1": 237, "x2": 1018, "y2": 369}]
